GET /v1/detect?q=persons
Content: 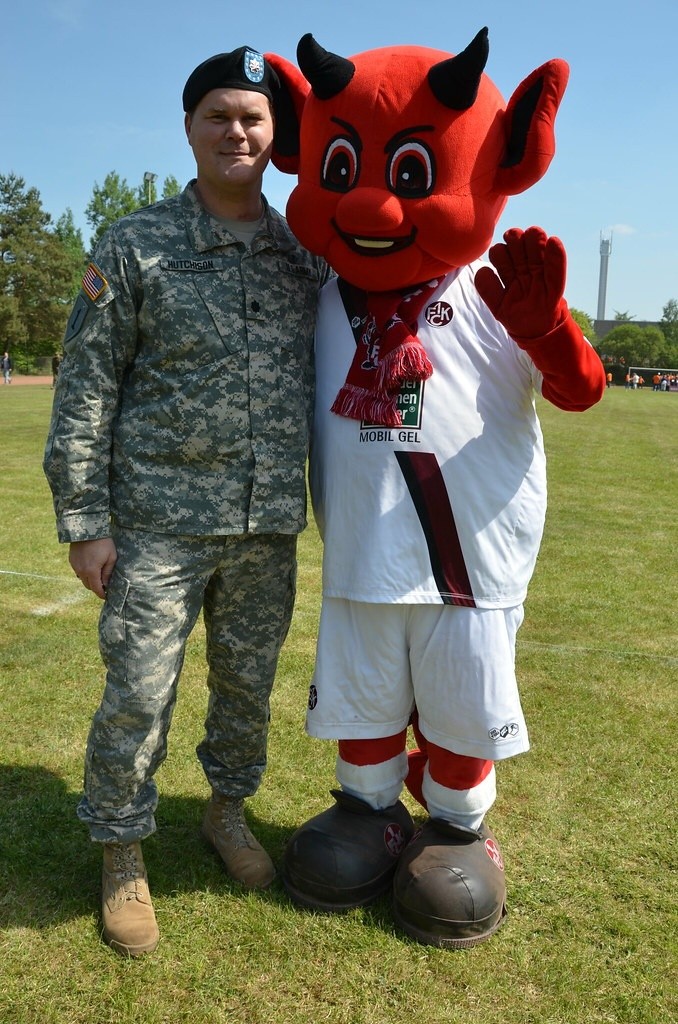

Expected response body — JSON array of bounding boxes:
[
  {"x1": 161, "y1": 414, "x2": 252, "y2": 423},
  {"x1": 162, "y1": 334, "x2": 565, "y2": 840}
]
[
  {"x1": 52, "y1": 352, "x2": 62, "y2": 387},
  {"x1": 607, "y1": 372, "x2": 613, "y2": 388},
  {"x1": 652, "y1": 372, "x2": 678, "y2": 391},
  {"x1": 625, "y1": 372, "x2": 645, "y2": 389},
  {"x1": 45, "y1": 47, "x2": 336, "y2": 955},
  {"x1": 1, "y1": 352, "x2": 13, "y2": 384}
]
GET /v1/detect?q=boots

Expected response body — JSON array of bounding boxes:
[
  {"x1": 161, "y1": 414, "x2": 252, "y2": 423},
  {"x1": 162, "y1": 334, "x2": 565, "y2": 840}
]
[
  {"x1": 202, "y1": 789, "x2": 276, "y2": 890},
  {"x1": 101, "y1": 837, "x2": 161, "y2": 956}
]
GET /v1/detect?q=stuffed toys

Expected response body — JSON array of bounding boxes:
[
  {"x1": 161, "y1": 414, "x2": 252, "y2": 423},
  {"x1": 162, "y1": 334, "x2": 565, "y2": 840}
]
[{"x1": 262, "y1": 29, "x2": 607, "y2": 947}]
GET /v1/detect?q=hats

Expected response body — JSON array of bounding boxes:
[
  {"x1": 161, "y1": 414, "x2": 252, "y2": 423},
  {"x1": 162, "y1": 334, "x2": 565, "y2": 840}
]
[{"x1": 180, "y1": 44, "x2": 292, "y2": 114}]
